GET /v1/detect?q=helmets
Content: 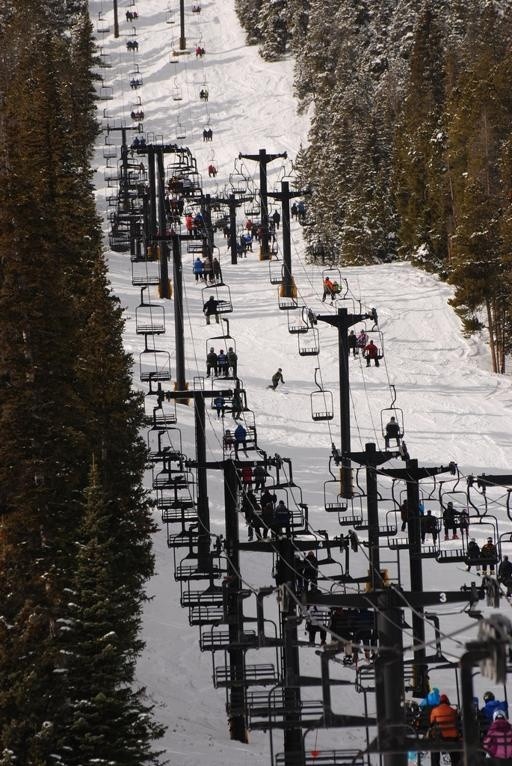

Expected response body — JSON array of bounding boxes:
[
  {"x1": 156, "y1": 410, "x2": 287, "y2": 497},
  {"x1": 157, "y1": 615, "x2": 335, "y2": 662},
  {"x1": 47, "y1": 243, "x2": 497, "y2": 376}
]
[
  {"x1": 492, "y1": 710, "x2": 506, "y2": 722},
  {"x1": 483, "y1": 691, "x2": 496, "y2": 703}
]
